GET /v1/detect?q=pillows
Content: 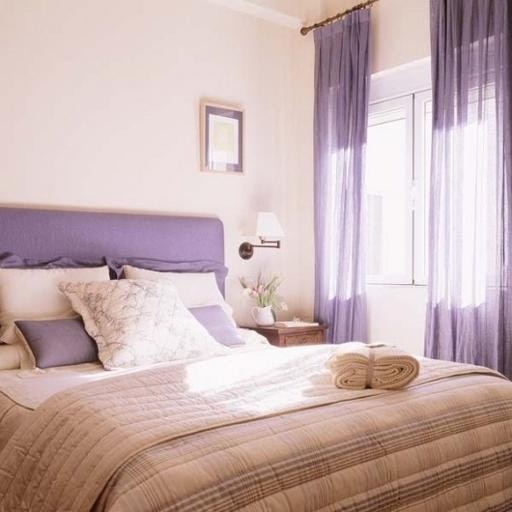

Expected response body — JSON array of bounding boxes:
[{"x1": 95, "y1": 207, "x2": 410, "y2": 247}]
[{"x1": 0, "y1": 253, "x2": 246, "y2": 376}]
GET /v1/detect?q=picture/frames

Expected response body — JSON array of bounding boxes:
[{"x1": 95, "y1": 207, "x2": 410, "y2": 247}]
[{"x1": 200, "y1": 99, "x2": 246, "y2": 175}]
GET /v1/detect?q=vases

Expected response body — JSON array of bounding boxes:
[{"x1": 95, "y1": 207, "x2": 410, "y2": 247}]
[{"x1": 251, "y1": 305, "x2": 275, "y2": 327}]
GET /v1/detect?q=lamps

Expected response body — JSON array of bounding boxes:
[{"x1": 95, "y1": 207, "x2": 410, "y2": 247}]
[{"x1": 239, "y1": 210, "x2": 285, "y2": 259}]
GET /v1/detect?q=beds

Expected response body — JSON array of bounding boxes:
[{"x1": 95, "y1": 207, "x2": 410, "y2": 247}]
[{"x1": 0, "y1": 206, "x2": 511, "y2": 510}]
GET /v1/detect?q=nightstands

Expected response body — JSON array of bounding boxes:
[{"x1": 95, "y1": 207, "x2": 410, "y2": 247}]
[{"x1": 239, "y1": 321, "x2": 329, "y2": 347}]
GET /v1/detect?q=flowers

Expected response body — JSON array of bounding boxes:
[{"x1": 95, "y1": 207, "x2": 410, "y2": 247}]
[{"x1": 239, "y1": 270, "x2": 289, "y2": 309}]
